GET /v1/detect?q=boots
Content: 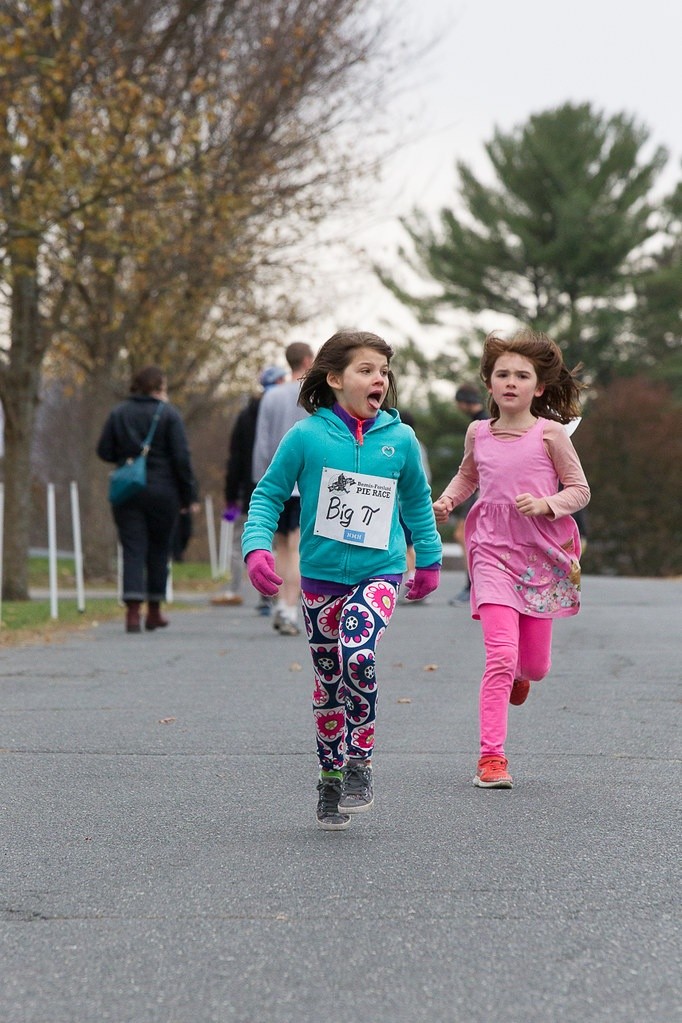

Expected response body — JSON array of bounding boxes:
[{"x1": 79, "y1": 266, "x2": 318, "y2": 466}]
[
  {"x1": 144, "y1": 600, "x2": 170, "y2": 629},
  {"x1": 125, "y1": 603, "x2": 142, "y2": 634}
]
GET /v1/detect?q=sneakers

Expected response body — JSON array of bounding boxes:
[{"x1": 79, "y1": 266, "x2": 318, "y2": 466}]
[
  {"x1": 510, "y1": 677, "x2": 530, "y2": 707},
  {"x1": 447, "y1": 588, "x2": 470, "y2": 607},
  {"x1": 338, "y1": 757, "x2": 375, "y2": 813},
  {"x1": 254, "y1": 596, "x2": 274, "y2": 616},
  {"x1": 316, "y1": 768, "x2": 352, "y2": 830},
  {"x1": 275, "y1": 606, "x2": 301, "y2": 639},
  {"x1": 473, "y1": 753, "x2": 514, "y2": 788}
]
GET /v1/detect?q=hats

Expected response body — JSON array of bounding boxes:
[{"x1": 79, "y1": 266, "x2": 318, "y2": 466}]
[{"x1": 258, "y1": 364, "x2": 285, "y2": 385}]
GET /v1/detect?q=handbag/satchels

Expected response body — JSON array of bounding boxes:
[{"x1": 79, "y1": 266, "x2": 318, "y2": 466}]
[{"x1": 109, "y1": 455, "x2": 148, "y2": 505}]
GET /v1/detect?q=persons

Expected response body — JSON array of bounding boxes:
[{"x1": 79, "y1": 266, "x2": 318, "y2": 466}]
[
  {"x1": 94, "y1": 365, "x2": 198, "y2": 632},
  {"x1": 242, "y1": 333, "x2": 444, "y2": 831},
  {"x1": 222, "y1": 367, "x2": 290, "y2": 616},
  {"x1": 435, "y1": 326, "x2": 591, "y2": 790},
  {"x1": 252, "y1": 340, "x2": 318, "y2": 635}
]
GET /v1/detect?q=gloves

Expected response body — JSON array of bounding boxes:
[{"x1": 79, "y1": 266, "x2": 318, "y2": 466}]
[
  {"x1": 246, "y1": 549, "x2": 282, "y2": 596},
  {"x1": 405, "y1": 569, "x2": 440, "y2": 599}
]
